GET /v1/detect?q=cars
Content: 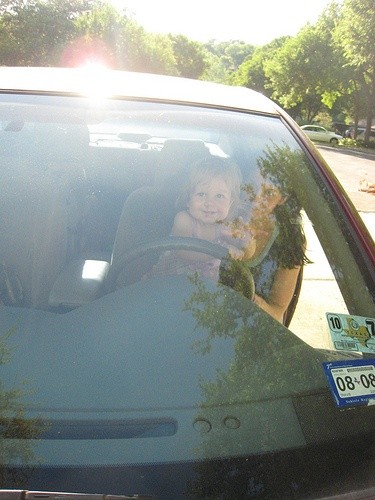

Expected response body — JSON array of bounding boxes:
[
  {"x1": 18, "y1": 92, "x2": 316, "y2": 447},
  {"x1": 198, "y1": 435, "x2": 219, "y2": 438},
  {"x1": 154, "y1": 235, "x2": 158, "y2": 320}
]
[
  {"x1": 331, "y1": 120, "x2": 374, "y2": 145},
  {"x1": 1, "y1": 64, "x2": 374, "y2": 496},
  {"x1": 298, "y1": 124, "x2": 343, "y2": 144}
]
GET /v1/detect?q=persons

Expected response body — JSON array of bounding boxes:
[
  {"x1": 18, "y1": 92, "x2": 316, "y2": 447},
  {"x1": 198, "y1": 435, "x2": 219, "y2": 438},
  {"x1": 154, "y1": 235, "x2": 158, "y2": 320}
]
[
  {"x1": 216, "y1": 148, "x2": 315, "y2": 323},
  {"x1": 162, "y1": 156, "x2": 257, "y2": 293}
]
